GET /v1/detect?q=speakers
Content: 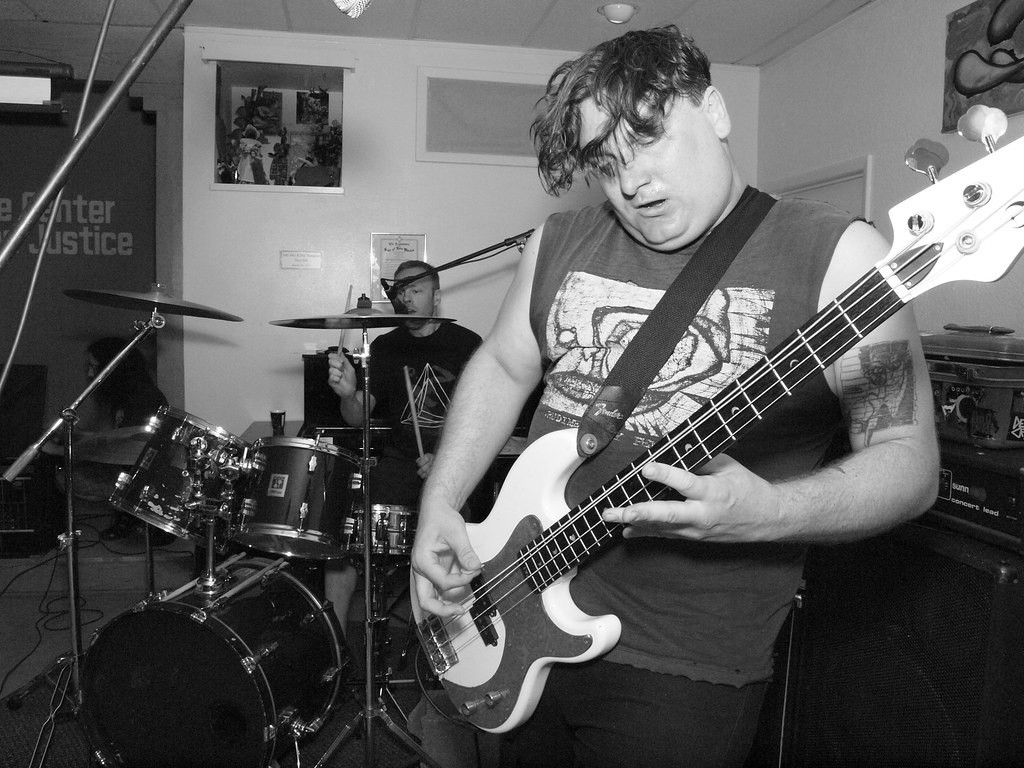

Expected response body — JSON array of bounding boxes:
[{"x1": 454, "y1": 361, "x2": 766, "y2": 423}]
[{"x1": 778, "y1": 517, "x2": 1024, "y2": 768}]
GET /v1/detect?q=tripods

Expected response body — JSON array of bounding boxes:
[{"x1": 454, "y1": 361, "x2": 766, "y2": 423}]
[
  {"x1": 2, "y1": 307, "x2": 165, "y2": 768},
  {"x1": 309, "y1": 322, "x2": 443, "y2": 768}
]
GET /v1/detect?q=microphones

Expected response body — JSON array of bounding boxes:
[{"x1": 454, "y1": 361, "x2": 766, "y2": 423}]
[{"x1": 381, "y1": 278, "x2": 409, "y2": 315}]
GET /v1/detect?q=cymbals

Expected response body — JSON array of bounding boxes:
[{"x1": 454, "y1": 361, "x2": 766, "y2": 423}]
[
  {"x1": 268, "y1": 306, "x2": 458, "y2": 329},
  {"x1": 58, "y1": 286, "x2": 244, "y2": 322}
]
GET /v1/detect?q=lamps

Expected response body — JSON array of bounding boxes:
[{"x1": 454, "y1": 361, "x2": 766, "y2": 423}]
[{"x1": 596, "y1": 2, "x2": 640, "y2": 24}]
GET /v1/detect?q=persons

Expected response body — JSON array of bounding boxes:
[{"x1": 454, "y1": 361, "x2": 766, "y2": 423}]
[
  {"x1": 323, "y1": 259, "x2": 486, "y2": 654},
  {"x1": 403, "y1": 23, "x2": 941, "y2": 768},
  {"x1": 82, "y1": 336, "x2": 186, "y2": 545}
]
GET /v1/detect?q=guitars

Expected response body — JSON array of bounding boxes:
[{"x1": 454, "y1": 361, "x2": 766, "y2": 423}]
[{"x1": 408, "y1": 102, "x2": 1024, "y2": 735}]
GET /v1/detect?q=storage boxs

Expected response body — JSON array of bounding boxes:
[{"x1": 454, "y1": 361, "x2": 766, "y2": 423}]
[{"x1": 916, "y1": 329, "x2": 1024, "y2": 450}]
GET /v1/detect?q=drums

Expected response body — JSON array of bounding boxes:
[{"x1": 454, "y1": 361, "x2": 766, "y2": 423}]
[
  {"x1": 227, "y1": 432, "x2": 370, "y2": 563},
  {"x1": 80, "y1": 554, "x2": 346, "y2": 768},
  {"x1": 108, "y1": 400, "x2": 254, "y2": 544},
  {"x1": 367, "y1": 501, "x2": 413, "y2": 556}
]
